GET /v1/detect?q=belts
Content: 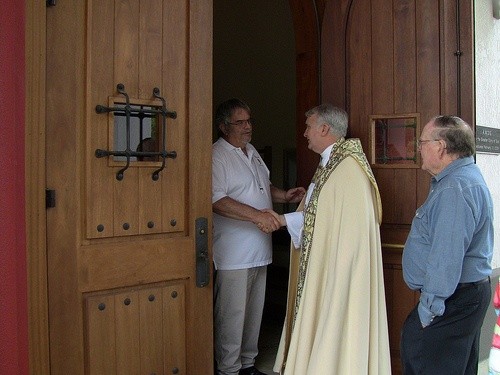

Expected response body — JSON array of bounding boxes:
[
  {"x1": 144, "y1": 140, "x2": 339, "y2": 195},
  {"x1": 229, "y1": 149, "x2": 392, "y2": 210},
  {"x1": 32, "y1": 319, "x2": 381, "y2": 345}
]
[{"x1": 454, "y1": 277, "x2": 488, "y2": 289}]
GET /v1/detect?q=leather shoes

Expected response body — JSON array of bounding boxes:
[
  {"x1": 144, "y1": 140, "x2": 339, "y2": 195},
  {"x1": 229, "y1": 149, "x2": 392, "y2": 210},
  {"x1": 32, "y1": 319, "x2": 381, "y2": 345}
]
[{"x1": 239, "y1": 366, "x2": 268, "y2": 375}]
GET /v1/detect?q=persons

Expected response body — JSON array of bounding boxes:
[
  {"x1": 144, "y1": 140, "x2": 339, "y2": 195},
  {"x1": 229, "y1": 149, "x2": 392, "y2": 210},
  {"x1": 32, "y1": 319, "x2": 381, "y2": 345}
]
[
  {"x1": 137, "y1": 137, "x2": 161, "y2": 164},
  {"x1": 258, "y1": 105, "x2": 392, "y2": 375},
  {"x1": 212, "y1": 98, "x2": 305, "y2": 375},
  {"x1": 399, "y1": 116, "x2": 494, "y2": 375}
]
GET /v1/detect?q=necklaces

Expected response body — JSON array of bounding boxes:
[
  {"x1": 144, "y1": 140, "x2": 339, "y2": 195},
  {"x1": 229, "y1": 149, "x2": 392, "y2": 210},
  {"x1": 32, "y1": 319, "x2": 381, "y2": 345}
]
[{"x1": 231, "y1": 144, "x2": 265, "y2": 195}]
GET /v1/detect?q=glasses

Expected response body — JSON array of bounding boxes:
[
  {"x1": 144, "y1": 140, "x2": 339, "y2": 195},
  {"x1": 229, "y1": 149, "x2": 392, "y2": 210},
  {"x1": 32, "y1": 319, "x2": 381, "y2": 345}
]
[
  {"x1": 417, "y1": 140, "x2": 439, "y2": 151},
  {"x1": 228, "y1": 118, "x2": 251, "y2": 126}
]
